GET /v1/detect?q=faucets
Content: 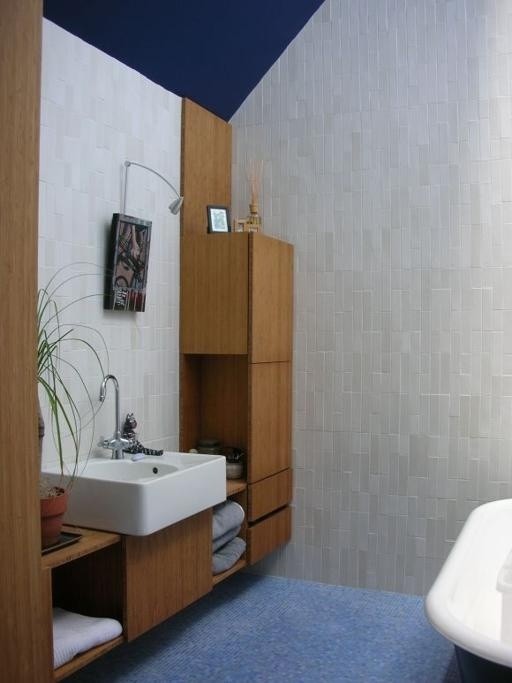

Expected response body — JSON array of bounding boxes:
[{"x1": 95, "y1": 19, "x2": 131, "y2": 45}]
[{"x1": 99, "y1": 373, "x2": 122, "y2": 439}]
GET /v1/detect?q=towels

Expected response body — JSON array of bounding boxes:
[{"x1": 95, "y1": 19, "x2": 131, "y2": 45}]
[
  {"x1": 52, "y1": 606, "x2": 123, "y2": 671},
  {"x1": 212, "y1": 501, "x2": 247, "y2": 575}
]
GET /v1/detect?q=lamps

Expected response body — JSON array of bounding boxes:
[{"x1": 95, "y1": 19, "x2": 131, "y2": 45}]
[{"x1": 123, "y1": 161, "x2": 184, "y2": 215}]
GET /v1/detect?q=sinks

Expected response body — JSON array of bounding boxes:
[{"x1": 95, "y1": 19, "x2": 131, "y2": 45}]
[{"x1": 40, "y1": 447, "x2": 226, "y2": 536}]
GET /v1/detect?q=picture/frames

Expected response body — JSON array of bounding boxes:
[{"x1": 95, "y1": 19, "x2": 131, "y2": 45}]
[
  {"x1": 104, "y1": 214, "x2": 152, "y2": 311},
  {"x1": 207, "y1": 206, "x2": 231, "y2": 233}
]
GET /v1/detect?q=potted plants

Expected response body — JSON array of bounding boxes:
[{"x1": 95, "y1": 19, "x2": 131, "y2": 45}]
[{"x1": 37, "y1": 249, "x2": 109, "y2": 549}]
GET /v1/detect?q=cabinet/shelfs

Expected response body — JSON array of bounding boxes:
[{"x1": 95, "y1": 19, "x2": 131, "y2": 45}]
[
  {"x1": 180, "y1": 97, "x2": 294, "y2": 564},
  {"x1": 41, "y1": 481, "x2": 247, "y2": 683}
]
